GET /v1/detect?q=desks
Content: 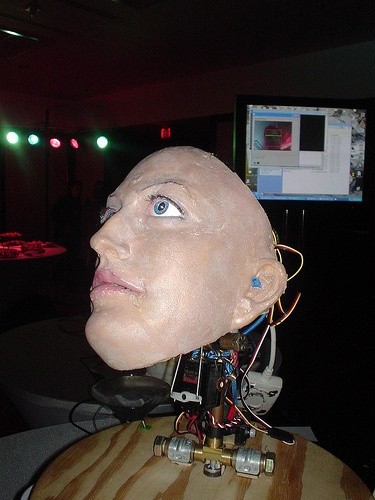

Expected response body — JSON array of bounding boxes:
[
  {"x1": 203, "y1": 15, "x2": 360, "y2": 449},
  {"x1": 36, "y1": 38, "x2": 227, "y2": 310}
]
[{"x1": 0, "y1": 232, "x2": 67, "y2": 299}]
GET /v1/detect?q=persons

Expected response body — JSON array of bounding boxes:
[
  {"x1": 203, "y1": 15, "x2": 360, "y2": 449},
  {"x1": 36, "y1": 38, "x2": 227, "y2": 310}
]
[
  {"x1": 86, "y1": 147, "x2": 288, "y2": 373},
  {"x1": 52, "y1": 181, "x2": 82, "y2": 282}
]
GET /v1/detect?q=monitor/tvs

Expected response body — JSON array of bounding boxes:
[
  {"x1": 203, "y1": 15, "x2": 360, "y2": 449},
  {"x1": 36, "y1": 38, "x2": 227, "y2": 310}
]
[{"x1": 232, "y1": 94, "x2": 373, "y2": 208}]
